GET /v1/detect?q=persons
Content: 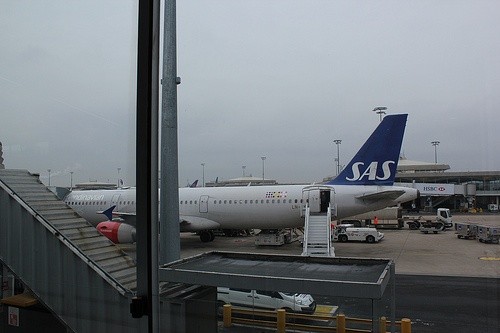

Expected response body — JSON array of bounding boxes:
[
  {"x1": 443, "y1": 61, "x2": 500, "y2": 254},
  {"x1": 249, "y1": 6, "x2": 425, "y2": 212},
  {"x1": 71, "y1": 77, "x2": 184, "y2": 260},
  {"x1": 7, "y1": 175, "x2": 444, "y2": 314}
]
[
  {"x1": 321, "y1": 191, "x2": 327, "y2": 212},
  {"x1": 374, "y1": 216, "x2": 378, "y2": 229}
]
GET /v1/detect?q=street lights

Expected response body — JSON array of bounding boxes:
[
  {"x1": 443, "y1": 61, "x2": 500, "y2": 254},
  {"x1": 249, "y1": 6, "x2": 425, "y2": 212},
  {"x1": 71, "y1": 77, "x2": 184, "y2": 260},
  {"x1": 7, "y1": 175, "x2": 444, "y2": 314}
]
[
  {"x1": 334, "y1": 139, "x2": 342, "y2": 175},
  {"x1": 70, "y1": 172, "x2": 73, "y2": 186},
  {"x1": 117, "y1": 168, "x2": 121, "y2": 187},
  {"x1": 48, "y1": 169, "x2": 51, "y2": 186},
  {"x1": 260, "y1": 157, "x2": 266, "y2": 180},
  {"x1": 201, "y1": 162, "x2": 204, "y2": 187},
  {"x1": 373, "y1": 106, "x2": 387, "y2": 121},
  {"x1": 431, "y1": 141, "x2": 440, "y2": 163}
]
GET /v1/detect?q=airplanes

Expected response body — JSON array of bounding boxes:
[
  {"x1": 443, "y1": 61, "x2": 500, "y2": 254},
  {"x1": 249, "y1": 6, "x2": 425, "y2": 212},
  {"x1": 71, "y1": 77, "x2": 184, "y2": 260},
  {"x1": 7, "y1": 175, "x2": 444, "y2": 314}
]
[{"x1": 65, "y1": 113, "x2": 420, "y2": 245}]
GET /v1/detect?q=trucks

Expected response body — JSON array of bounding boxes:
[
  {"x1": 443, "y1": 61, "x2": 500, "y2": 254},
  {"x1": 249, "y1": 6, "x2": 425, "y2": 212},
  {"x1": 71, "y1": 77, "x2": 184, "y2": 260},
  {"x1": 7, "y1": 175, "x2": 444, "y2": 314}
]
[
  {"x1": 333, "y1": 222, "x2": 384, "y2": 243},
  {"x1": 403, "y1": 208, "x2": 453, "y2": 230}
]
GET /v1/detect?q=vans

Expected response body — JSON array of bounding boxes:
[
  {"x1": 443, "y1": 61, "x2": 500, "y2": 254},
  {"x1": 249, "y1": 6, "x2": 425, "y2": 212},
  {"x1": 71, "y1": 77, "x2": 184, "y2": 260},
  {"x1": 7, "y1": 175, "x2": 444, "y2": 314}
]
[{"x1": 216, "y1": 286, "x2": 317, "y2": 320}]
